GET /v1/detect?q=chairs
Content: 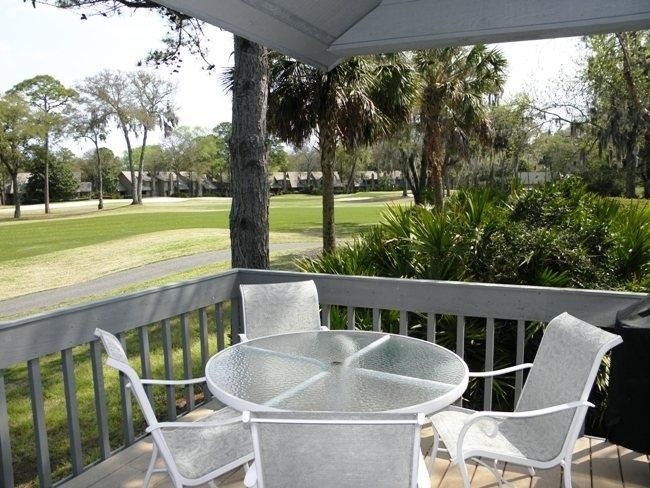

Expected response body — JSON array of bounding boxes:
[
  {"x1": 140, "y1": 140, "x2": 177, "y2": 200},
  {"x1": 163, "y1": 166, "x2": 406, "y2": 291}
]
[
  {"x1": 94, "y1": 327, "x2": 256, "y2": 488},
  {"x1": 428, "y1": 310, "x2": 624, "y2": 488},
  {"x1": 244, "y1": 410, "x2": 430, "y2": 488},
  {"x1": 237, "y1": 279, "x2": 356, "y2": 358}
]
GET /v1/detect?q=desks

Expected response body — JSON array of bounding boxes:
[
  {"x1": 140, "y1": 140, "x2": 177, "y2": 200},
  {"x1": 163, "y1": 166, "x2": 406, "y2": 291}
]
[{"x1": 204, "y1": 329, "x2": 471, "y2": 417}]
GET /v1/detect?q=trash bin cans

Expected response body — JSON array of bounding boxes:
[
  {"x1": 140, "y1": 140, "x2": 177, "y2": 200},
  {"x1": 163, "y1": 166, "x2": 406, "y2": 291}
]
[{"x1": 606, "y1": 292, "x2": 650, "y2": 455}]
